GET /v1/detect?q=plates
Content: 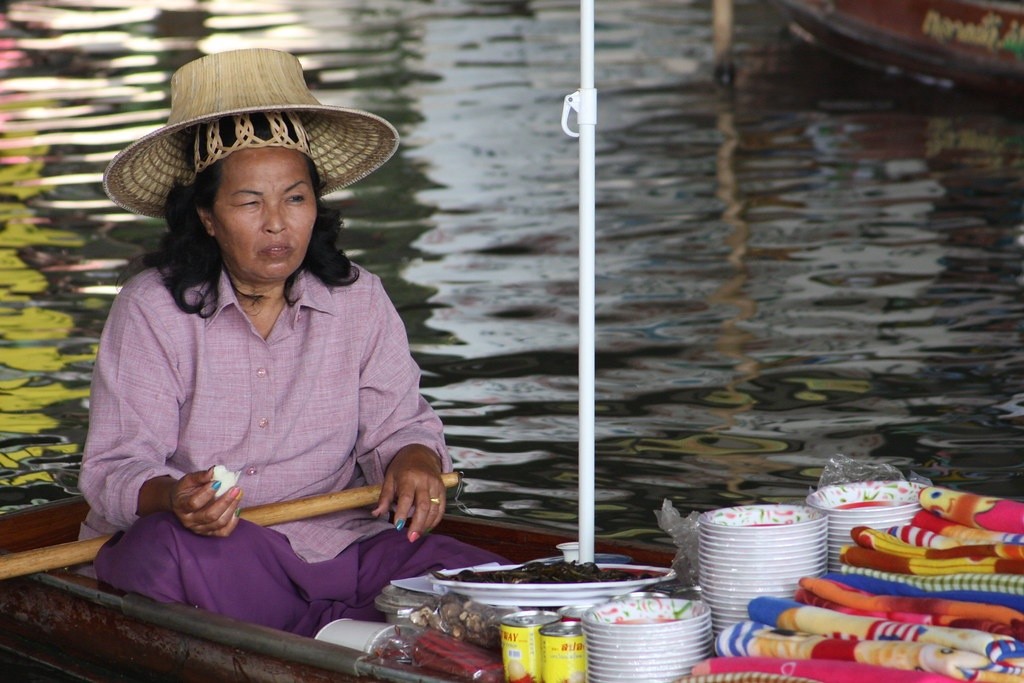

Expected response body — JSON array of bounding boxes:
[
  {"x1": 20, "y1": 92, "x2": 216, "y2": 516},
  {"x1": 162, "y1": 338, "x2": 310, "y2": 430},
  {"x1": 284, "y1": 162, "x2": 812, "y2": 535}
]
[
  {"x1": 806, "y1": 480, "x2": 930, "y2": 575},
  {"x1": 581, "y1": 598, "x2": 715, "y2": 683},
  {"x1": 697, "y1": 504, "x2": 829, "y2": 640},
  {"x1": 427, "y1": 562, "x2": 678, "y2": 607}
]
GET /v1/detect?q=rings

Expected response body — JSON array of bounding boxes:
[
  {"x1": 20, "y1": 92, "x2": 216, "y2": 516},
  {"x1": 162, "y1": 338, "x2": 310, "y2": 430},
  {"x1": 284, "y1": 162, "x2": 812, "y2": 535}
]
[{"x1": 430, "y1": 498, "x2": 440, "y2": 505}]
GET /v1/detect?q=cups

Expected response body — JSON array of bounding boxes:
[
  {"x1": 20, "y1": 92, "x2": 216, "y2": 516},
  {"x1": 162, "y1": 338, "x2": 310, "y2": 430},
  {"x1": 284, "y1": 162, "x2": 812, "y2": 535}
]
[
  {"x1": 314, "y1": 618, "x2": 417, "y2": 656},
  {"x1": 555, "y1": 541, "x2": 580, "y2": 563}
]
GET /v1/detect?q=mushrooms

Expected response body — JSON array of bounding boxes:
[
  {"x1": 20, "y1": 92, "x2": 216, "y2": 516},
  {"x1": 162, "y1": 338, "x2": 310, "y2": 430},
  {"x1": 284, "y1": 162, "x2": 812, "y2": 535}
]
[{"x1": 409, "y1": 594, "x2": 518, "y2": 650}]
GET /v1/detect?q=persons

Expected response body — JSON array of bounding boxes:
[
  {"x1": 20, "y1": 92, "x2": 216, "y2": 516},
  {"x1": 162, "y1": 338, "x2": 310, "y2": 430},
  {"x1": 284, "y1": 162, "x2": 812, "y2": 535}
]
[{"x1": 78, "y1": 49, "x2": 515, "y2": 639}]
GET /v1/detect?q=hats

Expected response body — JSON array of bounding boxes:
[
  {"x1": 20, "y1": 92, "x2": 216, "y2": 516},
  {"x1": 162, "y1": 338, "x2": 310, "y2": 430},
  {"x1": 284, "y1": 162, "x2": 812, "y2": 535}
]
[{"x1": 102, "y1": 49, "x2": 400, "y2": 219}]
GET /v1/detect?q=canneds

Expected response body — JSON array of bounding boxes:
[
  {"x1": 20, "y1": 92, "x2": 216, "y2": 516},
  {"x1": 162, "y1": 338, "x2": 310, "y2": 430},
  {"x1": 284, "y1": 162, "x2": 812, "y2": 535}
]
[{"x1": 498, "y1": 591, "x2": 670, "y2": 683}]
